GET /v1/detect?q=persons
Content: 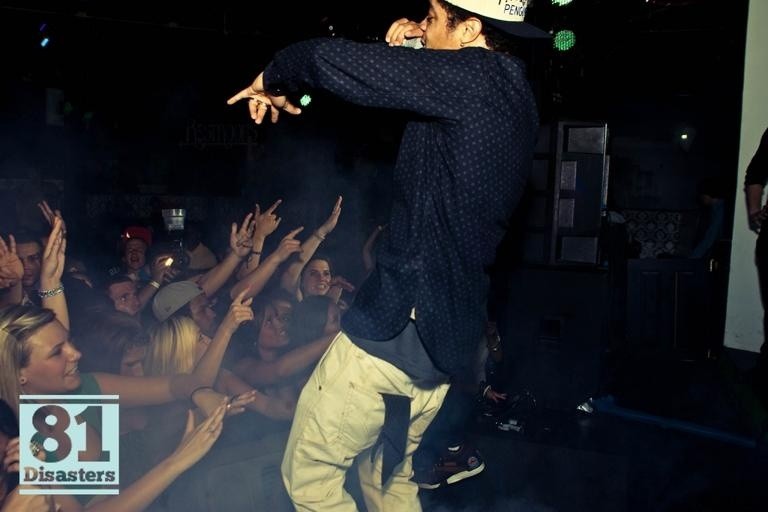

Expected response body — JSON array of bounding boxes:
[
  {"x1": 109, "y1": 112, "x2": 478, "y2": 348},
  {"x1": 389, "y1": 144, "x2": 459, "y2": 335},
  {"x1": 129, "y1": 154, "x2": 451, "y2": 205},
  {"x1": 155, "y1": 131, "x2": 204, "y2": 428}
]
[
  {"x1": 219, "y1": 1, "x2": 539, "y2": 511},
  {"x1": 1, "y1": 187, "x2": 522, "y2": 510},
  {"x1": 742, "y1": 125, "x2": 767, "y2": 375}
]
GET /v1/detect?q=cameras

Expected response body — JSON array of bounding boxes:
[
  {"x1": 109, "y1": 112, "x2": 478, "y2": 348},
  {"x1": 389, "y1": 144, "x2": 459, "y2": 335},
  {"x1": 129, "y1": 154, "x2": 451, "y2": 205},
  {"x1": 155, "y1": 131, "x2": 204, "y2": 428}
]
[{"x1": 160, "y1": 238, "x2": 189, "y2": 269}]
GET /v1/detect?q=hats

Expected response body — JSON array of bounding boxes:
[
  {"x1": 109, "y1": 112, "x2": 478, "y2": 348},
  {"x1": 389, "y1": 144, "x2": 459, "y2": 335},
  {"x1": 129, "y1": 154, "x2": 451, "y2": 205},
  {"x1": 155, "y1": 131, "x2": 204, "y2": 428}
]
[
  {"x1": 123, "y1": 227, "x2": 153, "y2": 246},
  {"x1": 153, "y1": 280, "x2": 205, "y2": 323},
  {"x1": 444, "y1": 0, "x2": 555, "y2": 40}
]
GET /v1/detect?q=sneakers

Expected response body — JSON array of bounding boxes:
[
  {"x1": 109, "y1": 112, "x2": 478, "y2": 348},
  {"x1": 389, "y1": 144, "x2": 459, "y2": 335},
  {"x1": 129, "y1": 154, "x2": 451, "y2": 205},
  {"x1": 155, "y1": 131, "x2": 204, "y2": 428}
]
[{"x1": 412, "y1": 442, "x2": 487, "y2": 489}]
